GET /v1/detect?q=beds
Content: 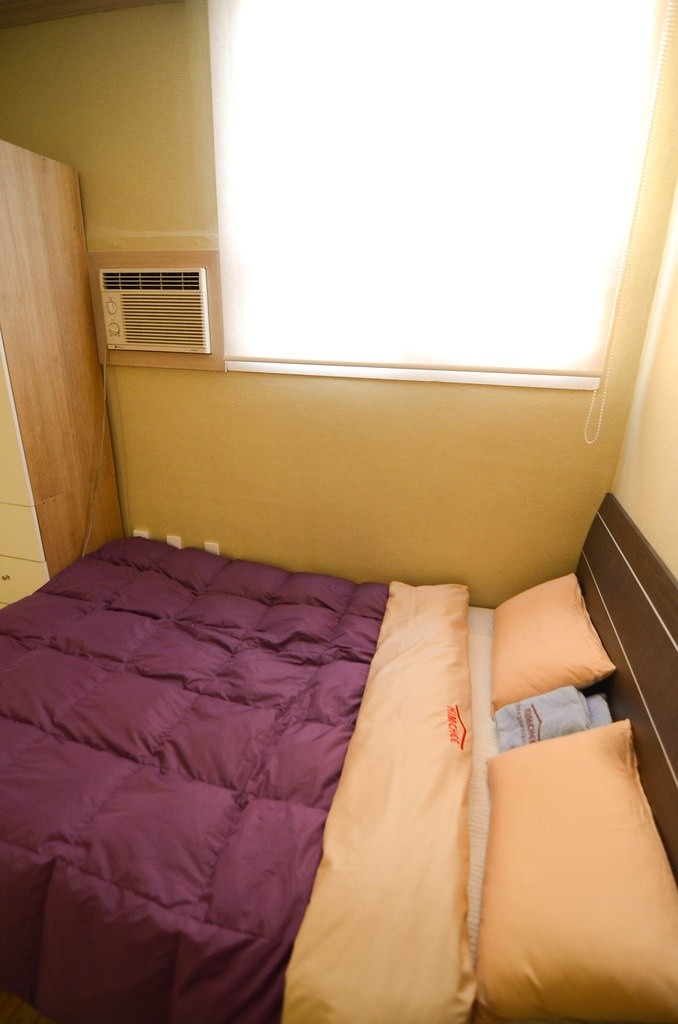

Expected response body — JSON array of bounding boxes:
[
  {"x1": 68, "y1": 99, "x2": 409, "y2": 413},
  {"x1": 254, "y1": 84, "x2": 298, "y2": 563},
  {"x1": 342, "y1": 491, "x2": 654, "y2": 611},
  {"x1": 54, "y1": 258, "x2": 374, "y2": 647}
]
[{"x1": 1, "y1": 492, "x2": 678, "y2": 1024}]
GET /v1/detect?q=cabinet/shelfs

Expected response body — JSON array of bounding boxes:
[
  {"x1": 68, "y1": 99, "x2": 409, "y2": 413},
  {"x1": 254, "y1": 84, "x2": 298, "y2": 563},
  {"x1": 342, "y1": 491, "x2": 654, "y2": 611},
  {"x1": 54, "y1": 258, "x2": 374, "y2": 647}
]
[{"x1": 0, "y1": 138, "x2": 123, "y2": 607}]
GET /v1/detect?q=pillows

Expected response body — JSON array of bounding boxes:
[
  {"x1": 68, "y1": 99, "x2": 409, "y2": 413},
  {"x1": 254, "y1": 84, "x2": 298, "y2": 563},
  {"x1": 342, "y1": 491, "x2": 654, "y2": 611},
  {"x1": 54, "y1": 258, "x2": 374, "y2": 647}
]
[
  {"x1": 475, "y1": 717, "x2": 678, "y2": 1023},
  {"x1": 492, "y1": 570, "x2": 616, "y2": 721}
]
